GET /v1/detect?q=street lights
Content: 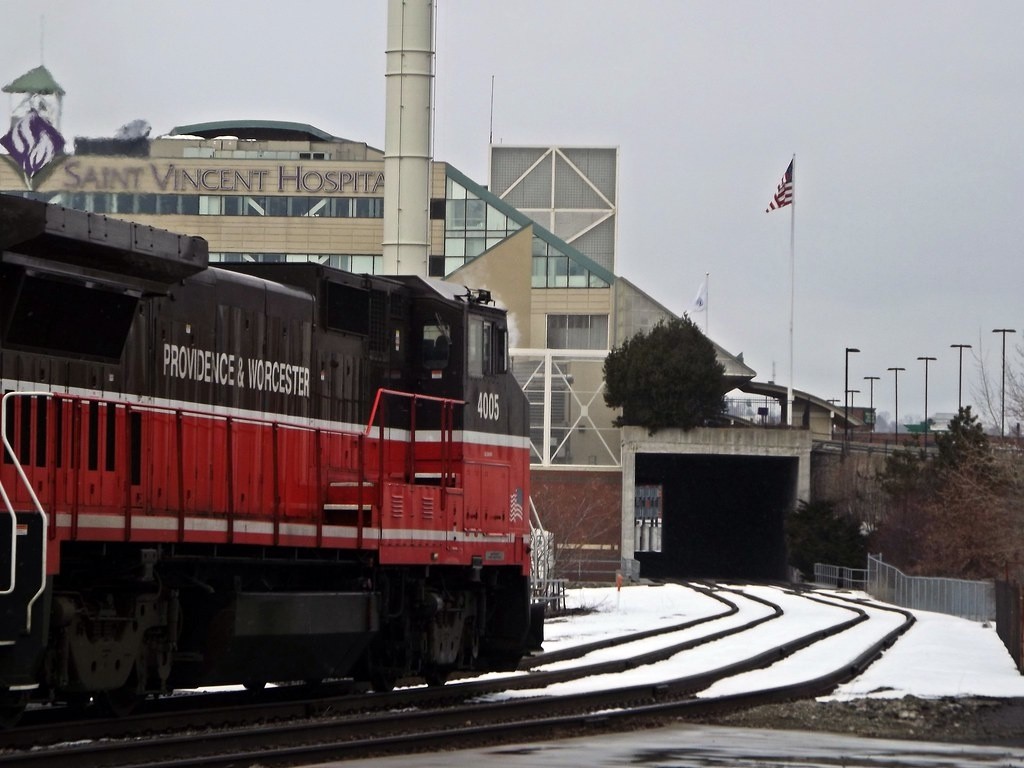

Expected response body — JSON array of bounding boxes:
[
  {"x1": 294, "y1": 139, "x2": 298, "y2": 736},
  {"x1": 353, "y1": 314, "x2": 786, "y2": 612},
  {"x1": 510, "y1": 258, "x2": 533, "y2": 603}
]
[
  {"x1": 843, "y1": 347, "x2": 862, "y2": 442},
  {"x1": 845, "y1": 390, "x2": 861, "y2": 439},
  {"x1": 991, "y1": 328, "x2": 1017, "y2": 437},
  {"x1": 863, "y1": 376, "x2": 880, "y2": 443},
  {"x1": 917, "y1": 356, "x2": 938, "y2": 453},
  {"x1": 887, "y1": 368, "x2": 905, "y2": 445},
  {"x1": 951, "y1": 343, "x2": 973, "y2": 411}
]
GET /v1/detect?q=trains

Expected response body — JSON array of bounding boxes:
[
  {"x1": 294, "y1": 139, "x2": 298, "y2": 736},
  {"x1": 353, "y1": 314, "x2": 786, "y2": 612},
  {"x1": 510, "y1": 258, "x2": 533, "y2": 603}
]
[{"x1": 0, "y1": 195, "x2": 553, "y2": 728}]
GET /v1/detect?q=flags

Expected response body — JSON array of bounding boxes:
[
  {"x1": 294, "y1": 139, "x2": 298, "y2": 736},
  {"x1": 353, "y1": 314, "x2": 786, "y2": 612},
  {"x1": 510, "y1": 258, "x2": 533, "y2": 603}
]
[
  {"x1": 765, "y1": 162, "x2": 794, "y2": 213},
  {"x1": 689, "y1": 281, "x2": 707, "y2": 313}
]
